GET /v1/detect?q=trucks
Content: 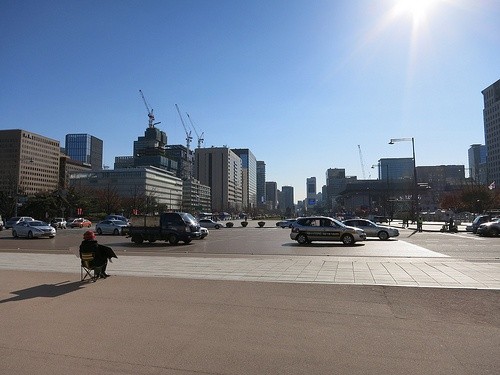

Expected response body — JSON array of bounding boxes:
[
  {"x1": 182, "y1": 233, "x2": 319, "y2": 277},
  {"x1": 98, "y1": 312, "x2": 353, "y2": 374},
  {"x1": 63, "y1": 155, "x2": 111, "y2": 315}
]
[{"x1": 129, "y1": 212, "x2": 208, "y2": 245}]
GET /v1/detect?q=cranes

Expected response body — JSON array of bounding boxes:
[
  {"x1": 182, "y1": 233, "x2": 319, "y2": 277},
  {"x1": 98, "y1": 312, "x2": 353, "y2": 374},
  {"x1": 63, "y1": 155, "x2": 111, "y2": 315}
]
[
  {"x1": 139, "y1": 89, "x2": 160, "y2": 128},
  {"x1": 175, "y1": 103, "x2": 192, "y2": 154},
  {"x1": 185, "y1": 112, "x2": 204, "y2": 150}
]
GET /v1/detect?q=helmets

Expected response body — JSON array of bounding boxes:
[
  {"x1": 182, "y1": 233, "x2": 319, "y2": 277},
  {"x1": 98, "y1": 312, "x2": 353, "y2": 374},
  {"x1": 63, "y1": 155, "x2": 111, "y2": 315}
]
[{"x1": 83, "y1": 231, "x2": 95, "y2": 239}]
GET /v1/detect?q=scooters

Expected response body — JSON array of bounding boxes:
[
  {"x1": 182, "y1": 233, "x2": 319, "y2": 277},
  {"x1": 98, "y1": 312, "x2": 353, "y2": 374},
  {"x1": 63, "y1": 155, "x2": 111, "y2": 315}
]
[{"x1": 439, "y1": 222, "x2": 458, "y2": 233}]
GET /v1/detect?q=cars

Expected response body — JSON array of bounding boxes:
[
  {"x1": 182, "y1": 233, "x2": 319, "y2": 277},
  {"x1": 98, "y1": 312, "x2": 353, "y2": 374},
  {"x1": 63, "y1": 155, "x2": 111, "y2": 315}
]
[
  {"x1": 276, "y1": 220, "x2": 303, "y2": 228},
  {"x1": 467, "y1": 214, "x2": 500, "y2": 236},
  {"x1": 49, "y1": 217, "x2": 67, "y2": 229},
  {"x1": 198, "y1": 219, "x2": 225, "y2": 229},
  {"x1": 289, "y1": 216, "x2": 367, "y2": 246},
  {"x1": 341, "y1": 219, "x2": 399, "y2": 241},
  {"x1": 69, "y1": 218, "x2": 92, "y2": 228},
  {"x1": 4, "y1": 217, "x2": 35, "y2": 229},
  {"x1": 95, "y1": 215, "x2": 130, "y2": 236},
  {"x1": 12, "y1": 221, "x2": 56, "y2": 240}
]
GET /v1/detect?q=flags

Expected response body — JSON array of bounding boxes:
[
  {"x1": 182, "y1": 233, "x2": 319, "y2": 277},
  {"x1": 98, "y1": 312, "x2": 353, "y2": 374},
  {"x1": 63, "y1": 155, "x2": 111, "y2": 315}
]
[{"x1": 488, "y1": 181, "x2": 495, "y2": 190}]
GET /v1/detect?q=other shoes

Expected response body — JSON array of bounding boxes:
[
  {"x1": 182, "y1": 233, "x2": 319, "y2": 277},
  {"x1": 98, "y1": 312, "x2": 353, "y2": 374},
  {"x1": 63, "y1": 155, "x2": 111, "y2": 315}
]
[{"x1": 97, "y1": 272, "x2": 110, "y2": 278}]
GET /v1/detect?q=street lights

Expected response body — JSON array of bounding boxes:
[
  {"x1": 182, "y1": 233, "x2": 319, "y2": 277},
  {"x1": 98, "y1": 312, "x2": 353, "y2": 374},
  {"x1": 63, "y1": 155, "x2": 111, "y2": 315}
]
[
  {"x1": 388, "y1": 138, "x2": 417, "y2": 224},
  {"x1": 371, "y1": 164, "x2": 390, "y2": 219}
]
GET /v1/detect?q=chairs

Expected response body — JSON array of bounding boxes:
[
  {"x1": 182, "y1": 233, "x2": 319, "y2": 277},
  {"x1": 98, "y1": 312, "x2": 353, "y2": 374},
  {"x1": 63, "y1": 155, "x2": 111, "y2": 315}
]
[{"x1": 80, "y1": 252, "x2": 101, "y2": 282}]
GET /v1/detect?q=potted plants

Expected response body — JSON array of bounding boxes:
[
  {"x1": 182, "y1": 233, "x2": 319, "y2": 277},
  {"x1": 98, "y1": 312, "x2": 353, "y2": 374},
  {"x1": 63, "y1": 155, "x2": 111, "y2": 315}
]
[{"x1": 226, "y1": 222, "x2": 234, "y2": 227}]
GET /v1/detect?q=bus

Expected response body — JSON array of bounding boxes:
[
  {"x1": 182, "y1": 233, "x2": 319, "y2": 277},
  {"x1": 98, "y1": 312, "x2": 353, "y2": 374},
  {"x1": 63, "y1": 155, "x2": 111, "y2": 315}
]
[{"x1": 197, "y1": 212, "x2": 231, "y2": 220}]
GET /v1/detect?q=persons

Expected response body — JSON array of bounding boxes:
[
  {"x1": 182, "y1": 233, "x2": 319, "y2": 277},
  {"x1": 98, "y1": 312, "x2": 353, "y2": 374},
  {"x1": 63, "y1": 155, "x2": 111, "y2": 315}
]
[
  {"x1": 51, "y1": 218, "x2": 57, "y2": 226},
  {"x1": 79, "y1": 230, "x2": 118, "y2": 278},
  {"x1": 446, "y1": 217, "x2": 453, "y2": 233},
  {"x1": 417, "y1": 215, "x2": 423, "y2": 232}
]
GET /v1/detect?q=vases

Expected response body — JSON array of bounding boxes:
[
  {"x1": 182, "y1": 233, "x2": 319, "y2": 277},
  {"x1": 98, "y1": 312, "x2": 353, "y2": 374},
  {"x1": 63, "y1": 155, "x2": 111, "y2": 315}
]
[
  {"x1": 241, "y1": 222, "x2": 248, "y2": 227},
  {"x1": 258, "y1": 222, "x2": 265, "y2": 227}
]
